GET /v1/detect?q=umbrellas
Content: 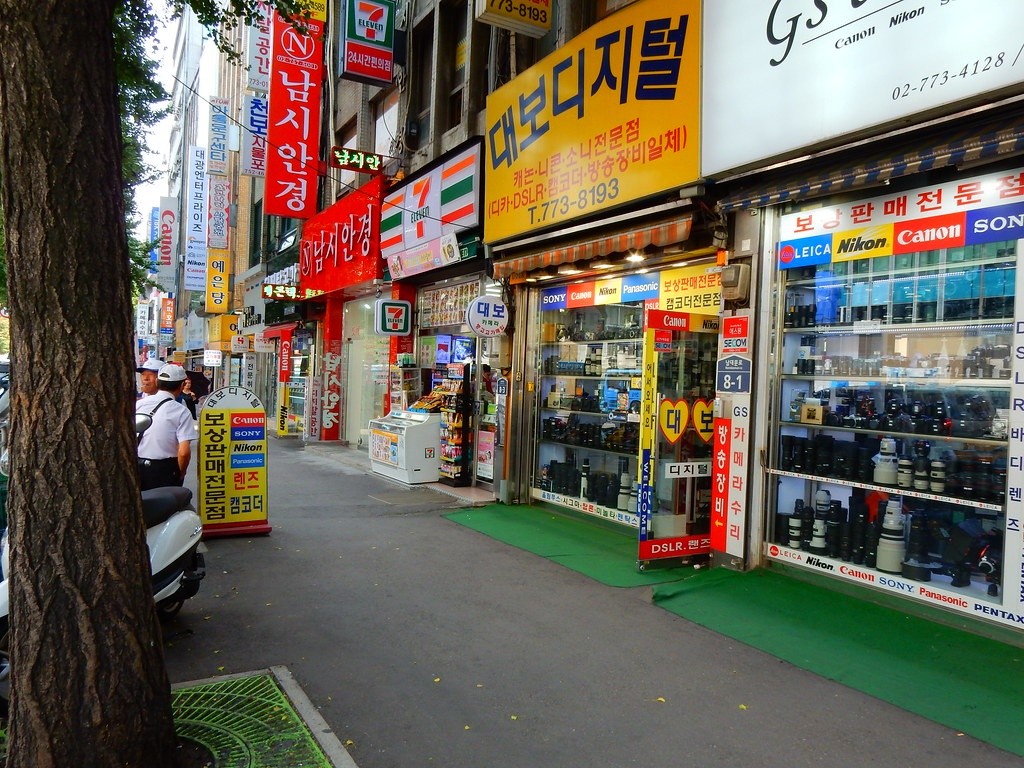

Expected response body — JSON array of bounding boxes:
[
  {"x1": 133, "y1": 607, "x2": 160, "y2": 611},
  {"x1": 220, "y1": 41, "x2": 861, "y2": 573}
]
[{"x1": 184, "y1": 370, "x2": 210, "y2": 398}]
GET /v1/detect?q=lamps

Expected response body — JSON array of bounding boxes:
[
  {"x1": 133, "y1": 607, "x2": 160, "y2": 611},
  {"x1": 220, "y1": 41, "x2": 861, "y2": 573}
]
[{"x1": 531, "y1": 243, "x2": 685, "y2": 279}]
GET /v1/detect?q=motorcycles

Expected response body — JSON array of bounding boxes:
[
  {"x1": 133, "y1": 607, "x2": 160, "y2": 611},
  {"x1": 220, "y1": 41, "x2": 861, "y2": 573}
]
[{"x1": 0, "y1": 413, "x2": 208, "y2": 720}]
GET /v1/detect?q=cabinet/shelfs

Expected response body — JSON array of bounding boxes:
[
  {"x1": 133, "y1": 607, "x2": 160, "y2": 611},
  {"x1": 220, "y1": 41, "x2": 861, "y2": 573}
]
[
  {"x1": 368, "y1": 363, "x2": 501, "y2": 484},
  {"x1": 534, "y1": 317, "x2": 1015, "y2": 514}
]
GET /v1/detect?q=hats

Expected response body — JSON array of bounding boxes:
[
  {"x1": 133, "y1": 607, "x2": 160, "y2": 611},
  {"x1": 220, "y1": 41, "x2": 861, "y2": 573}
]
[
  {"x1": 157, "y1": 362, "x2": 187, "y2": 381},
  {"x1": 136, "y1": 359, "x2": 165, "y2": 374}
]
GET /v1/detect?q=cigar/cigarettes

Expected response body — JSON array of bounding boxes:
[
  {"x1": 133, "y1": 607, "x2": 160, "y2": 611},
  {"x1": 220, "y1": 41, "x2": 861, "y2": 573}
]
[{"x1": 137, "y1": 385, "x2": 147, "y2": 389}]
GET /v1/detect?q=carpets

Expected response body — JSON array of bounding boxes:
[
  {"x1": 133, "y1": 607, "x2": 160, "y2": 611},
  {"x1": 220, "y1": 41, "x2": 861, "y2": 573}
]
[
  {"x1": 652, "y1": 564, "x2": 1024, "y2": 757},
  {"x1": 440, "y1": 503, "x2": 710, "y2": 588}
]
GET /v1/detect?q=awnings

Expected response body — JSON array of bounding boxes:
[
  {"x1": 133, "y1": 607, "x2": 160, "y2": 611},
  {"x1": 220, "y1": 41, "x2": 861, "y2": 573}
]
[{"x1": 492, "y1": 213, "x2": 692, "y2": 281}]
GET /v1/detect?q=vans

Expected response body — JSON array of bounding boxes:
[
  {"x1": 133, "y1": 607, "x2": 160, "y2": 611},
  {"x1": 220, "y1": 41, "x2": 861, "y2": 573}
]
[{"x1": 850, "y1": 386, "x2": 1006, "y2": 469}]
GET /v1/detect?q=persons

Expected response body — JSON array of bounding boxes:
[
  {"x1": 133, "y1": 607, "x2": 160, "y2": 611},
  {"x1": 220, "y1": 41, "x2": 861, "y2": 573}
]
[
  {"x1": 182, "y1": 379, "x2": 199, "y2": 421},
  {"x1": 135, "y1": 359, "x2": 165, "y2": 401},
  {"x1": 134, "y1": 364, "x2": 198, "y2": 490}
]
